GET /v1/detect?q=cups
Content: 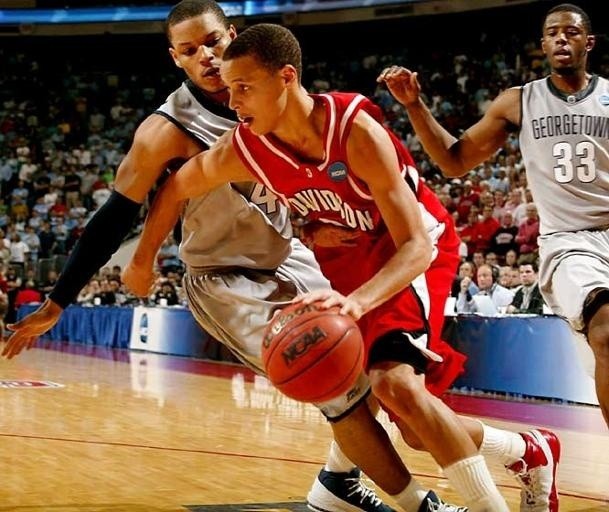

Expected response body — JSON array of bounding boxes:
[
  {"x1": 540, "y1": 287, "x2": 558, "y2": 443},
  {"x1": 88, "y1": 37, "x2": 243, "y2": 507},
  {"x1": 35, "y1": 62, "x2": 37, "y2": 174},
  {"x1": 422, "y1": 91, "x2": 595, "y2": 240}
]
[
  {"x1": 160, "y1": 298, "x2": 168, "y2": 308},
  {"x1": 93, "y1": 296, "x2": 101, "y2": 307}
]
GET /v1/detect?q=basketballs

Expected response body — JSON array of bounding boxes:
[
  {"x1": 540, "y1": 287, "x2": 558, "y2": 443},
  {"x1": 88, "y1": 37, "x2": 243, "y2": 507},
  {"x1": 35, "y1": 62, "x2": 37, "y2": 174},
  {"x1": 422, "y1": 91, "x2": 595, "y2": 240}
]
[{"x1": 260, "y1": 302, "x2": 364, "y2": 404}]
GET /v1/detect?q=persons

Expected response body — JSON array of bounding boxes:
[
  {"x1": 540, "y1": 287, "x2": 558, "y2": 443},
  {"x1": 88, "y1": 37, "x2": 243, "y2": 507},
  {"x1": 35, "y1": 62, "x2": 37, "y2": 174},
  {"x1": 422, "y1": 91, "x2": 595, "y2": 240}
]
[
  {"x1": 116, "y1": 19, "x2": 564, "y2": 512},
  {"x1": 2, "y1": 35, "x2": 189, "y2": 307},
  {"x1": 1, "y1": 2, "x2": 467, "y2": 511},
  {"x1": 301, "y1": 36, "x2": 554, "y2": 315},
  {"x1": 375, "y1": 2, "x2": 608, "y2": 427}
]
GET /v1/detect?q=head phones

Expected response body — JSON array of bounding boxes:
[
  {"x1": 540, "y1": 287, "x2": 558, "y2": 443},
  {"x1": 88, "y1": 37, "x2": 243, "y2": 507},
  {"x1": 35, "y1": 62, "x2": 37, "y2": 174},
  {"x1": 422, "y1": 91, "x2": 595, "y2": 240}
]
[{"x1": 491, "y1": 265, "x2": 500, "y2": 278}]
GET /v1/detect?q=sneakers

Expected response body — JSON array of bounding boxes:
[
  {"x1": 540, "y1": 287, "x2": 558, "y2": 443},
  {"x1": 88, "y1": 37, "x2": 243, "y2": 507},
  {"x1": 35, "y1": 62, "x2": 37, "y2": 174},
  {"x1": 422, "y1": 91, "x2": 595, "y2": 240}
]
[
  {"x1": 417, "y1": 489, "x2": 470, "y2": 512},
  {"x1": 306, "y1": 465, "x2": 398, "y2": 512},
  {"x1": 504, "y1": 428, "x2": 562, "y2": 512}
]
